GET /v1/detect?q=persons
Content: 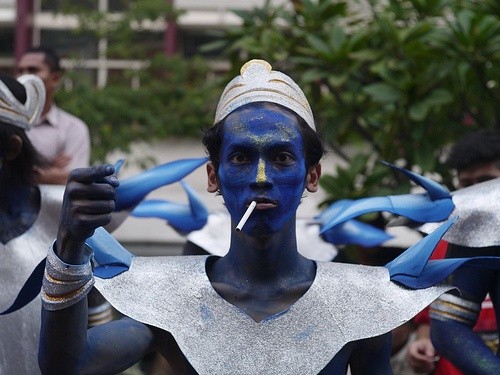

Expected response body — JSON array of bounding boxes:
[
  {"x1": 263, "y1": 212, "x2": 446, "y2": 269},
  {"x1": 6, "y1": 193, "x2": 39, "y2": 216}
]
[{"x1": 0, "y1": 46, "x2": 500, "y2": 375}]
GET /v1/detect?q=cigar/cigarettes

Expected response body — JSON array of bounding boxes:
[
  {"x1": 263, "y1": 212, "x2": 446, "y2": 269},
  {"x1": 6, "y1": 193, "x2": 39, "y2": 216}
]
[{"x1": 235, "y1": 200, "x2": 257, "y2": 232}]
